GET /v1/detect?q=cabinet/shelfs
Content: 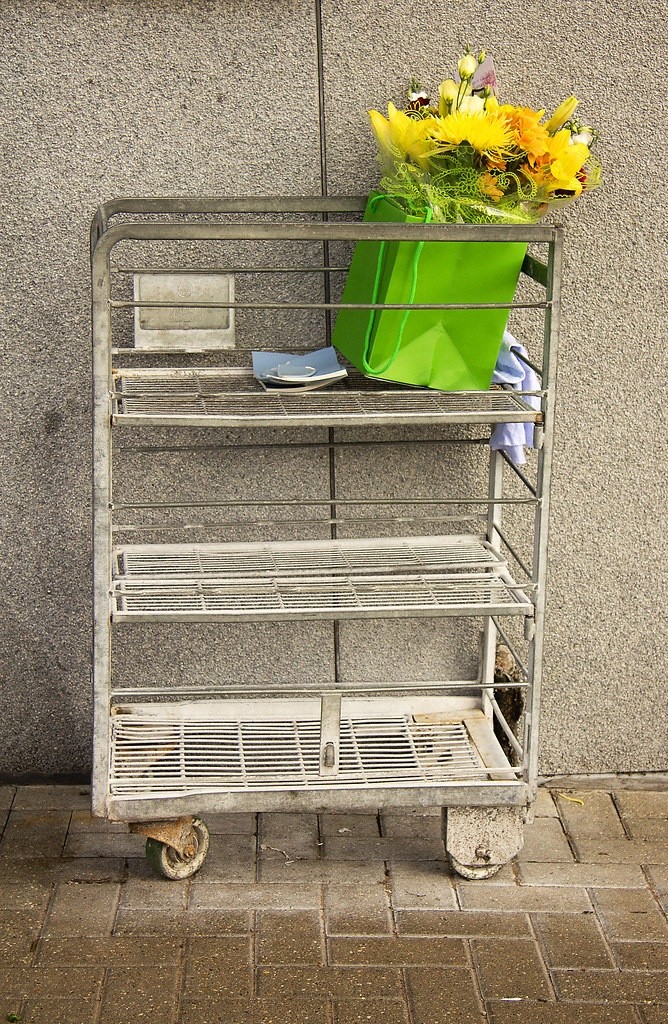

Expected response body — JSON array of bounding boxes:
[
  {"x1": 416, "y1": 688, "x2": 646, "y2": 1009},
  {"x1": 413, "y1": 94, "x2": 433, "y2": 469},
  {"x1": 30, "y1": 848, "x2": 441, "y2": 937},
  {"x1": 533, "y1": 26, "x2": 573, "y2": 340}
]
[{"x1": 88, "y1": 197, "x2": 562, "y2": 881}]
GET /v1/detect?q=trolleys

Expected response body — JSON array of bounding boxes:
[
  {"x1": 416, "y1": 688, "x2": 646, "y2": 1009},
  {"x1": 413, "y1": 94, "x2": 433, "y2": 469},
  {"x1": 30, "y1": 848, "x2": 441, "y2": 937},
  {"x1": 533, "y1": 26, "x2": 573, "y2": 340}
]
[{"x1": 92, "y1": 193, "x2": 564, "y2": 881}]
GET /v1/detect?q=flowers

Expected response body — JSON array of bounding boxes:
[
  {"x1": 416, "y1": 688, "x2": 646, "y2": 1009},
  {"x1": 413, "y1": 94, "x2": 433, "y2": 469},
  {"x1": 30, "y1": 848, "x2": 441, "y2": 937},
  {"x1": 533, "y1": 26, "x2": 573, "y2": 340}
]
[{"x1": 366, "y1": 47, "x2": 600, "y2": 229}]
